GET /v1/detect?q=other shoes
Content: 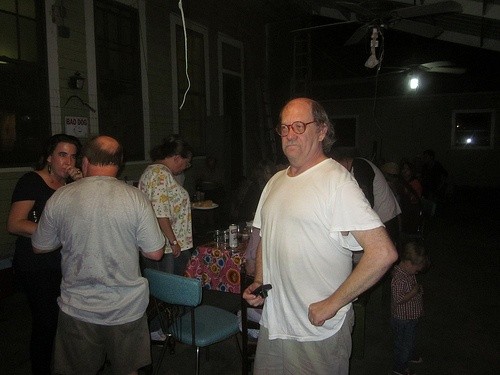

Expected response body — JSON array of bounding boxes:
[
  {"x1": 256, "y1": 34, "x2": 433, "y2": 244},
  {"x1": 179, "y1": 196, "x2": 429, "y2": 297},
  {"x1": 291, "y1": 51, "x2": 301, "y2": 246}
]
[
  {"x1": 408, "y1": 356, "x2": 422, "y2": 363},
  {"x1": 392, "y1": 370, "x2": 416, "y2": 375},
  {"x1": 150, "y1": 336, "x2": 179, "y2": 348}
]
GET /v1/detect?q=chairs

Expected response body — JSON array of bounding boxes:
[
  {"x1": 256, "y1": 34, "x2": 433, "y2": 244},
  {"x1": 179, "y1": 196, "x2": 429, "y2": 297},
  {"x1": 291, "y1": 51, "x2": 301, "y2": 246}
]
[
  {"x1": 240, "y1": 274, "x2": 265, "y2": 375},
  {"x1": 144, "y1": 268, "x2": 240, "y2": 375}
]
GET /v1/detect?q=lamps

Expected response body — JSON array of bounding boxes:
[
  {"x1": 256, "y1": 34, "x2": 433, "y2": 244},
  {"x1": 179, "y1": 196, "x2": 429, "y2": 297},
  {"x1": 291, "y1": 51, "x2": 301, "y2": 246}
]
[
  {"x1": 71, "y1": 73, "x2": 85, "y2": 89},
  {"x1": 405, "y1": 70, "x2": 423, "y2": 93},
  {"x1": 365, "y1": 27, "x2": 379, "y2": 69}
]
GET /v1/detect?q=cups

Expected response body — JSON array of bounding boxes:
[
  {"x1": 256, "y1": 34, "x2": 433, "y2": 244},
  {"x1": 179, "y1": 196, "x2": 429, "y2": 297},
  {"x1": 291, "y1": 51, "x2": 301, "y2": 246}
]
[
  {"x1": 240, "y1": 227, "x2": 250, "y2": 237},
  {"x1": 214, "y1": 229, "x2": 228, "y2": 243},
  {"x1": 30, "y1": 211, "x2": 40, "y2": 223}
]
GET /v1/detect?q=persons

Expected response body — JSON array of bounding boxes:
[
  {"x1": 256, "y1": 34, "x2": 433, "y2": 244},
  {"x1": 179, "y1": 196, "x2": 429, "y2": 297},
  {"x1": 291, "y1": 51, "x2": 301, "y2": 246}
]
[
  {"x1": 8, "y1": 134, "x2": 83, "y2": 375},
  {"x1": 391, "y1": 240, "x2": 425, "y2": 375},
  {"x1": 333, "y1": 149, "x2": 449, "y2": 305},
  {"x1": 138, "y1": 135, "x2": 193, "y2": 349},
  {"x1": 242, "y1": 97, "x2": 398, "y2": 375},
  {"x1": 32, "y1": 136, "x2": 165, "y2": 375}
]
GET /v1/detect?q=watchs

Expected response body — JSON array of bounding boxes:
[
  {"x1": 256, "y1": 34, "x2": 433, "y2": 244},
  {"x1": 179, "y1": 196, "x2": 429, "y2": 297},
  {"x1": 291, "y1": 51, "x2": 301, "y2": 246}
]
[{"x1": 168, "y1": 239, "x2": 178, "y2": 245}]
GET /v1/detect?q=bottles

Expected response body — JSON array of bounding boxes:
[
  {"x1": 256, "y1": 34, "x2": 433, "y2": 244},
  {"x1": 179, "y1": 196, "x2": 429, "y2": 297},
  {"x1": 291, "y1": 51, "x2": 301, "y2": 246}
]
[{"x1": 192, "y1": 186, "x2": 205, "y2": 202}]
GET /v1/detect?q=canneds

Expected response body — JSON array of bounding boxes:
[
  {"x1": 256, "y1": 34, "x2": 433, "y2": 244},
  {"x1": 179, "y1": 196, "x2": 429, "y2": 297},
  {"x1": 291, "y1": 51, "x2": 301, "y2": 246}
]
[{"x1": 229, "y1": 224, "x2": 238, "y2": 248}]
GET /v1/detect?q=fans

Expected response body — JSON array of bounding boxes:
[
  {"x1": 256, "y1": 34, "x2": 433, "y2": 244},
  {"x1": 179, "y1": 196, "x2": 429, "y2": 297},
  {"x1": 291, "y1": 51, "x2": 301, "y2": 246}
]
[
  {"x1": 369, "y1": 60, "x2": 469, "y2": 82},
  {"x1": 290, "y1": 0, "x2": 467, "y2": 44}
]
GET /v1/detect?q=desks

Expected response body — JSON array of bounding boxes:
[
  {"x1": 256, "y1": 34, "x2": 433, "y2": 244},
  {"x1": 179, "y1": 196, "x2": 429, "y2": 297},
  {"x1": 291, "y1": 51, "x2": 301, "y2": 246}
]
[{"x1": 181, "y1": 240, "x2": 260, "y2": 375}]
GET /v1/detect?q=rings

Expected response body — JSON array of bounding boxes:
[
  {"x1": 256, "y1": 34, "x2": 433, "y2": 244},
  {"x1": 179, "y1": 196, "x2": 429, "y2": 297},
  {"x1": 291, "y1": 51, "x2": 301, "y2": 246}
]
[{"x1": 77, "y1": 170, "x2": 78, "y2": 174}]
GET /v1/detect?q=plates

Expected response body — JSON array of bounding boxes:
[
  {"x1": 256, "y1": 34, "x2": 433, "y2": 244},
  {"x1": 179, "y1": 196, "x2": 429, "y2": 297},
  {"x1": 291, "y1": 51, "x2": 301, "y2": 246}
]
[{"x1": 192, "y1": 202, "x2": 219, "y2": 210}]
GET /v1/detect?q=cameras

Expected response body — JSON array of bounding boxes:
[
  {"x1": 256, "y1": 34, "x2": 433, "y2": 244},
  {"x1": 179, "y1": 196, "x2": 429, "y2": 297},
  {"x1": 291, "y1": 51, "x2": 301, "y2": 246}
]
[{"x1": 251, "y1": 284, "x2": 272, "y2": 298}]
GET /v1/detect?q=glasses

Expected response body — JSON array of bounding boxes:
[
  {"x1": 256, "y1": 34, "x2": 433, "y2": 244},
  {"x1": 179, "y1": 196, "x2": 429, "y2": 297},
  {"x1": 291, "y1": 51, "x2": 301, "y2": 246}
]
[{"x1": 275, "y1": 121, "x2": 317, "y2": 137}]
